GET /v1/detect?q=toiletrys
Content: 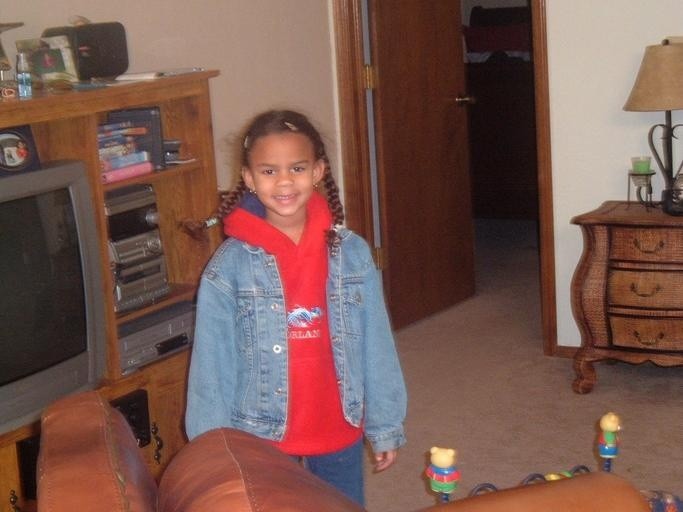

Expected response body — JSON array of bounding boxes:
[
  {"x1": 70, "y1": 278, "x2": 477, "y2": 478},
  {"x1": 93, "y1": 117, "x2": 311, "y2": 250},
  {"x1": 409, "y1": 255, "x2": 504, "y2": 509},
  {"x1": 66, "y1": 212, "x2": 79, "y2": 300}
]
[{"x1": 15, "y1": 50, "x2": 31, "y2": 98}]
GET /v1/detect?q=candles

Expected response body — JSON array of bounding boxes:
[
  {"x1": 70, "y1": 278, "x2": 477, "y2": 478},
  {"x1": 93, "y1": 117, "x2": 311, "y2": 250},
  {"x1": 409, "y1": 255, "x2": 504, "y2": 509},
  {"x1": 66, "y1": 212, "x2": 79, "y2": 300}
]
[{"x1": 631, "y1": 155, "x2": 652, "y2": 174}]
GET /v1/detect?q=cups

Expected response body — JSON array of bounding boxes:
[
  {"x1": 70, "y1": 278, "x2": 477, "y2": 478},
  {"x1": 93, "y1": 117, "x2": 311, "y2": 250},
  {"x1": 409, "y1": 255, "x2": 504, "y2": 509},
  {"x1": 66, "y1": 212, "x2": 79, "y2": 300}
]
[{"x1": 632, "y1": 156, "x2": 651, "y2": 186}]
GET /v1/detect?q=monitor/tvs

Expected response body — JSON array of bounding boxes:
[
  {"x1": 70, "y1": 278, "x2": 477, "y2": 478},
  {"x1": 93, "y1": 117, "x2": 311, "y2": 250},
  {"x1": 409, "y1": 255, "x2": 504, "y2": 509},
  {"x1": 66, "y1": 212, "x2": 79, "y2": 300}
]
[{"x1": 0, "y1": 159, "x2": 107, "y2": 436}]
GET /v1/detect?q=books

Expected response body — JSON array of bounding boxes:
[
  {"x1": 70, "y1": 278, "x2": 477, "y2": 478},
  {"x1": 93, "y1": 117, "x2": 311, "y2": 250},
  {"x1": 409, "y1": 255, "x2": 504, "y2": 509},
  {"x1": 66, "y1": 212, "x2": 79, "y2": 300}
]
[
  {"x1": 95, "y1": 119, "x2": 156, "y2": 185},
  {"x1": 114, "y1": 69, "x2": 176, "y2": 82}
]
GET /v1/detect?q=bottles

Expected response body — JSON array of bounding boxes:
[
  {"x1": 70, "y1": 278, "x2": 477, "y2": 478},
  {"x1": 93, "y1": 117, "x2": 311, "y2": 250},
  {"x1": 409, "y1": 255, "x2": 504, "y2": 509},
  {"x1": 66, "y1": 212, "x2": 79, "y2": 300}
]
[{"x1": 16, "y1": 53, "x2": 33, "y2": 96}]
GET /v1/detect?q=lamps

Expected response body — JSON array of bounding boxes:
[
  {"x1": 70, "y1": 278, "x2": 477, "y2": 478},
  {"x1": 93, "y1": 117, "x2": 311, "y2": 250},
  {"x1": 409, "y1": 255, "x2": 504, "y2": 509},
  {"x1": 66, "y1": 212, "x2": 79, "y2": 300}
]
[{"x1": 622, "y1": 44, "x2": 683, "y2": 218}]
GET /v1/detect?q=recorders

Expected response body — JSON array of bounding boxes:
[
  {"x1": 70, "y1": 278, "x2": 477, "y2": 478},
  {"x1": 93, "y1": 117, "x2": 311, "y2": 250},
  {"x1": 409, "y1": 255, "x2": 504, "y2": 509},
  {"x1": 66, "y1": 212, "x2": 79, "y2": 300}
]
[{"x1": 42, "y1": 22, "x2": 128, "y2": 82}]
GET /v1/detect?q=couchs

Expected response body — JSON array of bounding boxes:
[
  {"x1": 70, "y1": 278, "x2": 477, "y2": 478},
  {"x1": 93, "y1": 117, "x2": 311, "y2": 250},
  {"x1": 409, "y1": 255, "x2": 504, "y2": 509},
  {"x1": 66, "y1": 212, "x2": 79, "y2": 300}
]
[{"x1": 37, "y1": 391, "x2": 660, "y2": 512}]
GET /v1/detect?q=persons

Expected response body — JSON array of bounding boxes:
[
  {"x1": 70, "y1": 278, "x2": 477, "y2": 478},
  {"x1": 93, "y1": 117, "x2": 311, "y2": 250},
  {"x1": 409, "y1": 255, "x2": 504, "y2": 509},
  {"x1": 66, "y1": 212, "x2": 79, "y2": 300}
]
[
  {"x1": 178, "y1": 108, "x2": 410, "y2": 512},
  {"x1": 40, "y1": 52, "x2": 59, "y2": 68},
  {"x1": 4, "y1": 147, "x2": 18, "y2": 165},
  {"x1": 15, "y1": 139, "x2": 28, "y2": 159}
]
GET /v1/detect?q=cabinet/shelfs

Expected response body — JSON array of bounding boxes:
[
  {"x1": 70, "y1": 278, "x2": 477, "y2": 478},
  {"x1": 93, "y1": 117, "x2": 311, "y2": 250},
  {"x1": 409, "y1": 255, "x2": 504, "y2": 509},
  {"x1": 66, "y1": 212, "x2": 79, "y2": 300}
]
[
  {"x1": 571, "y1": 200, "x2": 683, "y2": 393},
  {"x1": 0, "y1": 70, "x2": 226, "y2": 511}
]
